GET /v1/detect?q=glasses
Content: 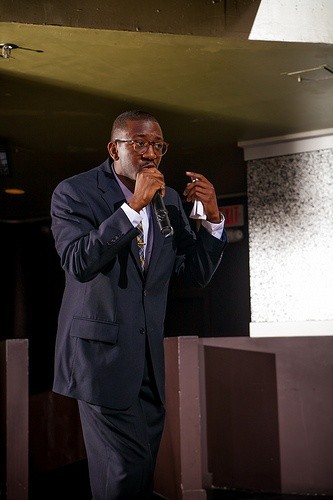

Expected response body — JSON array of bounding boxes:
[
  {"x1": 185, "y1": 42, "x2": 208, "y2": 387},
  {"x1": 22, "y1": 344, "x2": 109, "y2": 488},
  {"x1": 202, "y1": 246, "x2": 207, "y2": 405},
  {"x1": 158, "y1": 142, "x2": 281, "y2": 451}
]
[{"x1": 114, "y1": 138, "x2": 168, "y2": 156}]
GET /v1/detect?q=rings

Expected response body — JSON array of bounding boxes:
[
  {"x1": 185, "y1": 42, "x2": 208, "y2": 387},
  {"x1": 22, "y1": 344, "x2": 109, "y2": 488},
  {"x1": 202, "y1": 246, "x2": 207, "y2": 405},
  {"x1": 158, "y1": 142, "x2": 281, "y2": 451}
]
[{"x1": 161, "y1": 185, "x2": 165, "y2": 190}]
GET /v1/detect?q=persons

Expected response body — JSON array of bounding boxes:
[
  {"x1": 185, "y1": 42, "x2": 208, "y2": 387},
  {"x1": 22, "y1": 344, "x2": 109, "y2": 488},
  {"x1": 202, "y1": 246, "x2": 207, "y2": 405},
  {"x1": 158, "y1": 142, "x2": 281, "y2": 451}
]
[{"x1": 51, "y1": 109, "x2": 227, "y2": 500}]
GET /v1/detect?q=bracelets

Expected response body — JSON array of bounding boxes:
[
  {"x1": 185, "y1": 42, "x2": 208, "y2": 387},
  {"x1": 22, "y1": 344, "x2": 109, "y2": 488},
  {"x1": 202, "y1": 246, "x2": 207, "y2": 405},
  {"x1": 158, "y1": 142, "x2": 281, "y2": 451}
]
[{"x1": 207, "y1": 212, "x2": 224, "y2": 224}]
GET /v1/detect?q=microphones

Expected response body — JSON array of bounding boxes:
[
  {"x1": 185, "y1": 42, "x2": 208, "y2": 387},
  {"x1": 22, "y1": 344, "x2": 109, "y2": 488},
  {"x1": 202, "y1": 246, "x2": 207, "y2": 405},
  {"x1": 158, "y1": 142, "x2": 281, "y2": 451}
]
[{"x1": 137, "y1": 161, "x2": 173, "y2": 237}]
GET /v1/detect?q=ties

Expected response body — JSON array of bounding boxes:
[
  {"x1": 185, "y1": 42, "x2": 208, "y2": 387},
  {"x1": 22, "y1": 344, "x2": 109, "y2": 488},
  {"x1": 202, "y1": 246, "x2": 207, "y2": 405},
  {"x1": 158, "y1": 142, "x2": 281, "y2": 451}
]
[{"x1": 137, "y1": 221, "x2": 144, "y2": 267}]
